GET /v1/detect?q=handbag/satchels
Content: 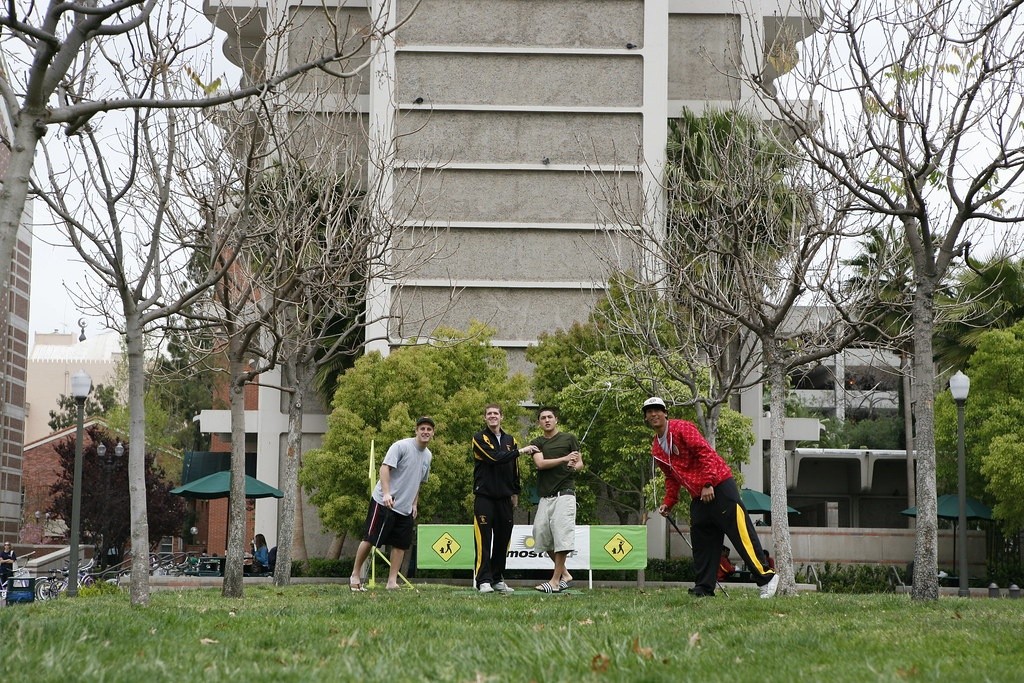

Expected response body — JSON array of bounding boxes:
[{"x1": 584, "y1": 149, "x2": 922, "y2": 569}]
[{"x1": 10, "y1": 550, "x2": 18, "y2": 575}]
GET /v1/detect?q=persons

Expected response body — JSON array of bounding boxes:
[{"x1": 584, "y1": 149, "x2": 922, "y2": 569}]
[
  {"x1": 642, "y1": 396, "x2": 780, "y2": 600},
  {"x1": 529, "y1": 408, "x2": 584, "y2": 594},
  {"x1": 0, "y1": 540, "x2": 17, "y2": 591},
  {"x1": 471, "y1": 405, "x2": 540, "y2": 593},
  {"x1": 762, "y1": 549, "x2": 775, "y2": 572},
  {"x1": 349, "y1": 417, "x2": 435, "y2": 592},
  {"x1": 247, "y1": 534, "x2": 269, "y2": 577},
  {"x1": 716, "y1": 545, "x2": 743, "y2": 583}
]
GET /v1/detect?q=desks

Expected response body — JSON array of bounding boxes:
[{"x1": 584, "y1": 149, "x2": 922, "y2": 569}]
[{"x1": 199, "y1": 555, "x2": 254, "y2": 577}]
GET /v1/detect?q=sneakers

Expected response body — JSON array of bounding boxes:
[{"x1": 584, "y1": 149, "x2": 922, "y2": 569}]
[
  {"x1": 492, "y1": 581, "x2": 515, "y2": 592},
  {"x1": 759, "y1": 574, "x2": 780, "y2": 598},
  {"x1": 480, "y1": 582, "x2": 494, "y2": 592}
]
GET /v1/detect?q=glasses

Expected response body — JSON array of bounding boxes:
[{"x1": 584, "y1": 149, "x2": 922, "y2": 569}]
[{"x1": 4, "y1": 544, "x2": 10, "y2": 546}]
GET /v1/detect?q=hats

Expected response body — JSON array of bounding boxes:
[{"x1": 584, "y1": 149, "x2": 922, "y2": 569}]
[
  {"x1": 416, "y1": 417, "x2": 436, "y2": 427},
  {"x1": 642, "y1": 396, "x2": 667, "y2": 411}
]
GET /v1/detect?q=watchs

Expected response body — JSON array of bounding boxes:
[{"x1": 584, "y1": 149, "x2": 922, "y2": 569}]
[{"x1": 704, "y1": 482, "x2": 711, "y2": 488}]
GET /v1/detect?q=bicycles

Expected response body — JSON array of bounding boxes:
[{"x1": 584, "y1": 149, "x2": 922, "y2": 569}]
[{"x1": 0, "y1": 557, "x2": 97, "y2": 602}]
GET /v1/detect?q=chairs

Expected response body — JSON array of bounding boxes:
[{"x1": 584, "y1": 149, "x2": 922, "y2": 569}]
[{"x1": 257, "y1": 547, "x2": 277, "y2": 577}]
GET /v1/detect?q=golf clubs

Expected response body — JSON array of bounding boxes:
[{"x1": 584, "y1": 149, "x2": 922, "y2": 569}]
[
  {"x1": 566, "y1": 380, "x2": 613, "y2": 471},
  {"x1": 360, "y1": 495, "x2": 395, "y2": 592},
  {"x1": 660, "y1": 507, "x2": 730, "y2": 598}
]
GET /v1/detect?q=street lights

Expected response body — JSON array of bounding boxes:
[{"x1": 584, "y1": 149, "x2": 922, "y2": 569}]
[
  {"x1": 97, "y1": 442, "x2": 125, "y2": 571},
  {"x1": 63, "y1": 368, "x2": 94, "y2": 597},
  {"x1": 34, "y1": 510, "x2": 50, "y2": 544},
  {"x1": 189, "y1": 527, "x2": 199, "y2": 554},
  {"x1": 947, "y1": 369, "x2": 972, "y2": 598}
]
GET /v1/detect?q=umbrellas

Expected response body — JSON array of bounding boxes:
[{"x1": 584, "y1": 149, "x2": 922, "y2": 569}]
[
  {"x1": 899, "y1": 493, "x2": 995, "y2": 576},
  {"x1": 168, "y1": 467, "x2": 285, "y2": 557},
  {"x1": 739, "y1": 487, "x2": 798, "y2": 515}
]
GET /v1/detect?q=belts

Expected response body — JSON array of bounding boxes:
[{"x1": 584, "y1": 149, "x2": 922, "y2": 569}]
[{"x1": 544, "y1": 492, "x2": 574, "y2": 498}]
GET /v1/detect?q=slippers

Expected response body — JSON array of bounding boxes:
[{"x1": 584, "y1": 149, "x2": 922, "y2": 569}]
[
  {"x1": 349, "y1": 579, "x2": 363, "y2": 592},
  {"x1": 534, "y1": 582, "x2": 561, "y2": 593},
  {"x1": 559, "y1": 580, "x2": 573, "y2": 590}
]
